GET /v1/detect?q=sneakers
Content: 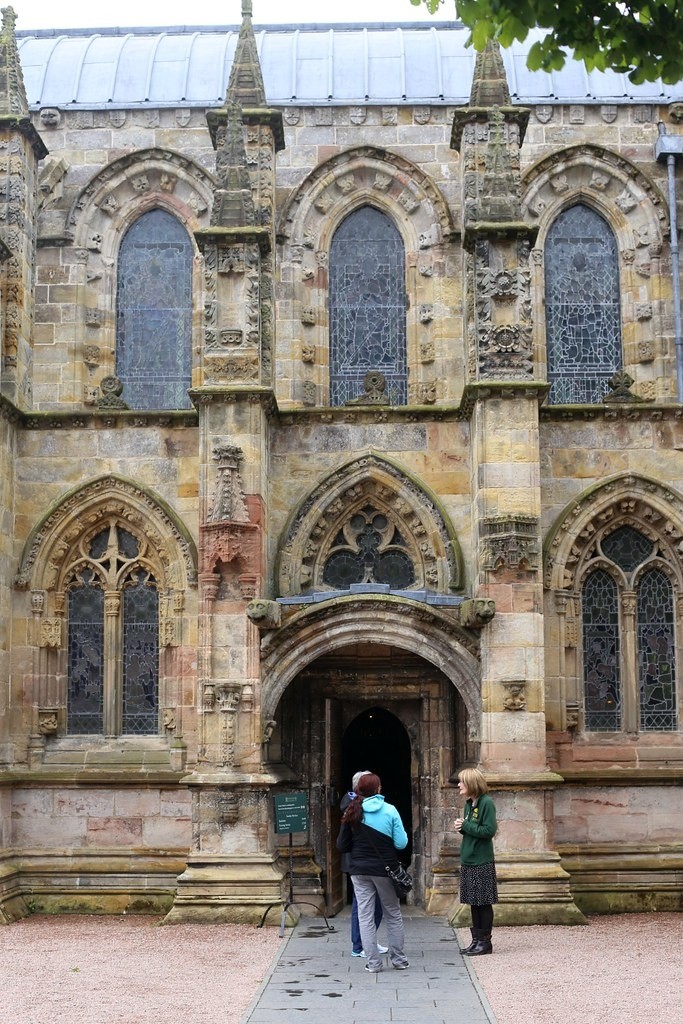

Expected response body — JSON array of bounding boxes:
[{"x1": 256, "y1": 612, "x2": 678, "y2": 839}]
[
  {"x1": 364, "y1": 963, "x2": 381, "y2": 972},
  {"x1": 375, "y1": 944, "x2": 389, "y2": 953},
  {"x1": 351, "y1": 950, "x2": 367, "y2": 958},
  {"x1": 395, "y1": 959, "x2": 410, "y2": 969}
]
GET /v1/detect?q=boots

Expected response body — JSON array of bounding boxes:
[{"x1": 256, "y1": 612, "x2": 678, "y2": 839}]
[
  {"x1": 466, "y1": 929, "x2": 492, "y2": 955},
  {"x1": 458, "y1": 927, "x2": 478, "y2": 953}
]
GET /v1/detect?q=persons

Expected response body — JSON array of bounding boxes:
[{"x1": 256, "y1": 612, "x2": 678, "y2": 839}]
[
  {"x1": 340, "y1": 770, "x2": 390, "y2": 957},
  {"x1": 336, "y1": 774, "x2": 409, "y2": 973},
  {"x1": 454, "y1": 767, "x2": 499, "y2": 956}
]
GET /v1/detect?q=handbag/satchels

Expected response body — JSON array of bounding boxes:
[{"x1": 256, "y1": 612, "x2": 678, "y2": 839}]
[{"x1": 386, "y1": 863, "x2": 412, "y2": 898}]
